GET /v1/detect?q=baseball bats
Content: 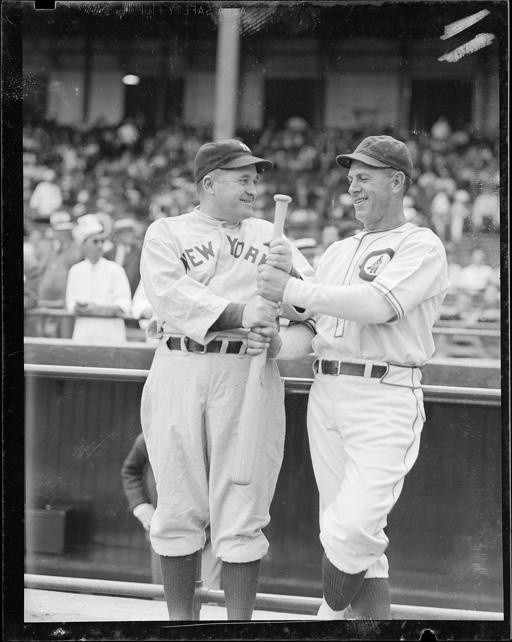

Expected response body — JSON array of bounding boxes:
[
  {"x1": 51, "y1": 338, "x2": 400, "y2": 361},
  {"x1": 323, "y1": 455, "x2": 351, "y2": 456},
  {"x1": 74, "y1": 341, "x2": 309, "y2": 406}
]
[{"x1": 230, "y1": 193, "x2": 291, "y2": 484}]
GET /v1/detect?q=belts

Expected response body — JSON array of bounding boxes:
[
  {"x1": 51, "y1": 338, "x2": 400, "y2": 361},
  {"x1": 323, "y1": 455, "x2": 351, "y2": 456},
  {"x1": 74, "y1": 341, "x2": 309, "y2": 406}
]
[
  {"x1": 168, "y1": 337, "x2": 240, "y2": 355},
  {"x1": 317, "y1": 360, "x2": 386, "y2": 380}
]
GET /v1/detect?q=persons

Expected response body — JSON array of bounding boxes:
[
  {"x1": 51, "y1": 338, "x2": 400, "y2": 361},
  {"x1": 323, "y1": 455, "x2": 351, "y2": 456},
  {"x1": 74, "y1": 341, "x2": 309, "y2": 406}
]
[
  {"x1": 139, "y1": 139, "x2": 312, "y2": 620},
  {"x1": 120, "y1": 429, "x2": 222, "y2": 601},
  {"x1": 245, "y1": 135, "x2": 451, "y2": 622},
  {"x1": 23, "y1": 107, "x2": 500, "y2": 344}
]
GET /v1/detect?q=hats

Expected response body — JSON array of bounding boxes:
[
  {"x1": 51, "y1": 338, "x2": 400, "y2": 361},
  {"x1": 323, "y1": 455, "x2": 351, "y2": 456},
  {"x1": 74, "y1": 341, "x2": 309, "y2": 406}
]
[
  {"x1": 195, "y1": 139, "x2": 274, "y2": 183},
  {"x1": 336, "y1": 135, "x2": 414, "y2": 178}
]
